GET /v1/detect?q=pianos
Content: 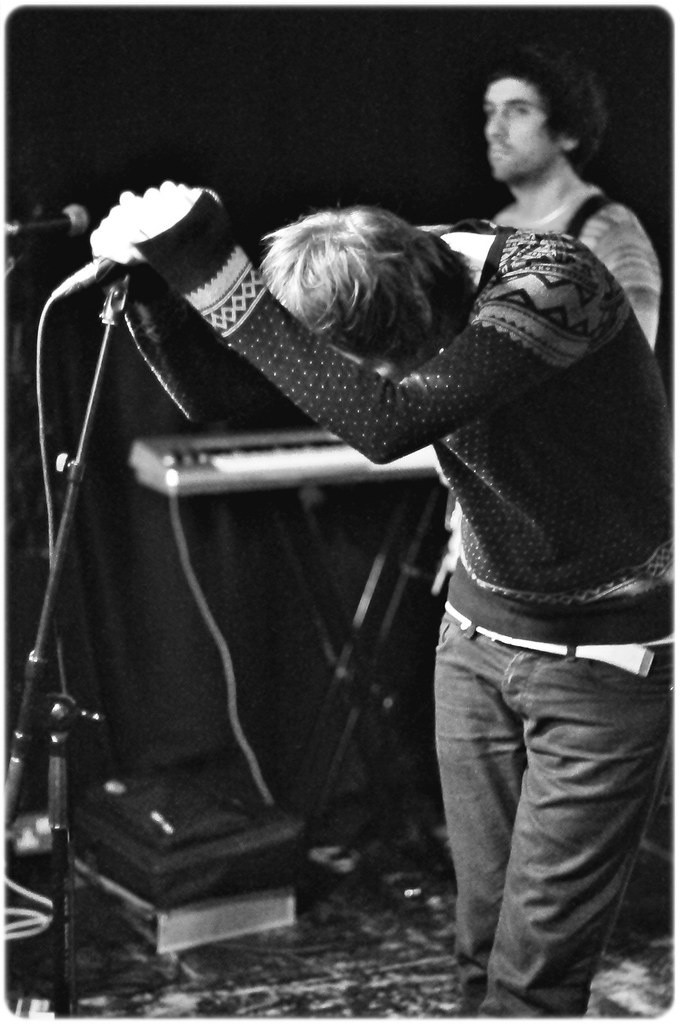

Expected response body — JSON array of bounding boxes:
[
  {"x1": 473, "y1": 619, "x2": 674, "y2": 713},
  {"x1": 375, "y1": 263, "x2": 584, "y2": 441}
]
[{"x1": 130, "y1": 413, "x2": 457, "y2": 908}]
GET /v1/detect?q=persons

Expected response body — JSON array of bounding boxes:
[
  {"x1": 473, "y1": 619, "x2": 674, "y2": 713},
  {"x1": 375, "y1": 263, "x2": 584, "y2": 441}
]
[
  {"x1": 461, "y1": 52, "x2": 662, "y2": 347},
  {"x1": 85, "y1": 181, "x2": 672, "y2": 1019}
]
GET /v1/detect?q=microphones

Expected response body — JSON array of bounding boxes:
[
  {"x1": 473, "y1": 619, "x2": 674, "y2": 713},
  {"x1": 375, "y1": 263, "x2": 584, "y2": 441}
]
[
  {"x1": 6, "y1": 203, "x2": 89, "y2": 240},
  {"x1": 50, "y1": 186, "x2": 224, "y2": 301}
]
